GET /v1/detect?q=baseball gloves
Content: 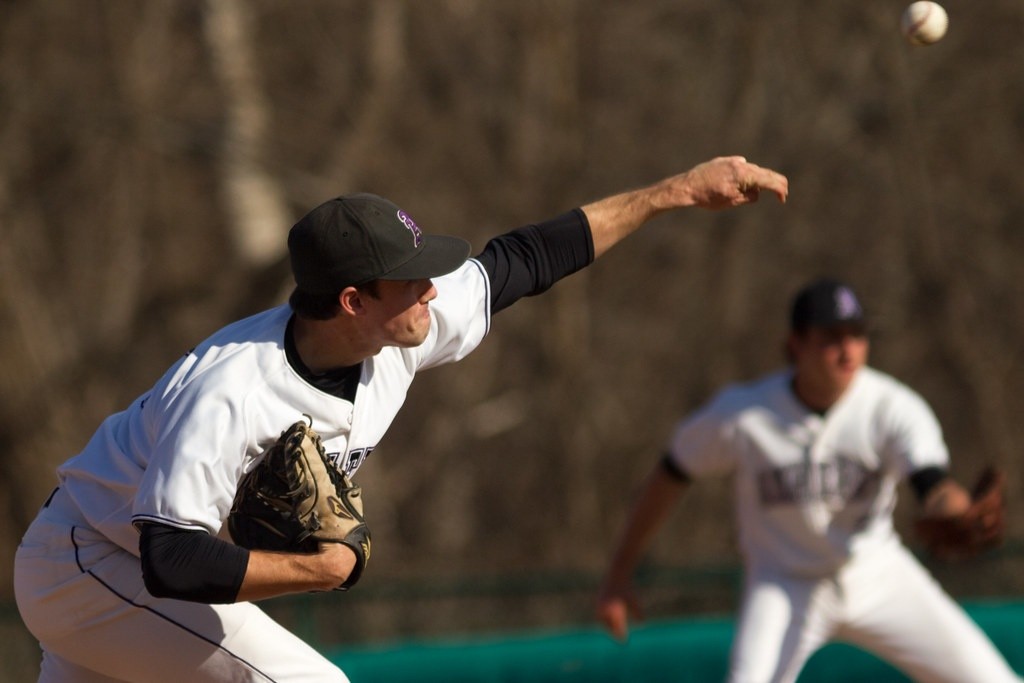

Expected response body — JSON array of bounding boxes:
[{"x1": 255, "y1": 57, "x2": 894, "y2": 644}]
[
  {"x1": 923, "y1": 460, "x2": 1007, "y2": 549},
  {"x1": 224, "y1": 418, "x2": 374, "y2": 594}
]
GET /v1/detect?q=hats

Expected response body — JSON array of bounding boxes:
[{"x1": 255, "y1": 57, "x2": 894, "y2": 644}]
[
  {"x1": 790, "y1": 277, "x2": 866, "y2": 329},
  {"x1": 288, "y1": 194, "x2": 471, "y2": 281}
]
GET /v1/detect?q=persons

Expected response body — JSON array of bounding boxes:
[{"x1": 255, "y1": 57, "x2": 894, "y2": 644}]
[
  {"x1": 13, "y1": 155, "x2": 789, "y2": 683},
  {"x1": 599, "y1": 280, "x2": 1020, "y2": 683}
]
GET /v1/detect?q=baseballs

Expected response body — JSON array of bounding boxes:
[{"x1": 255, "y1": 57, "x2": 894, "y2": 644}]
[{"x1": 897, "y1": 0, "x2": 950, "y2": 45}]
